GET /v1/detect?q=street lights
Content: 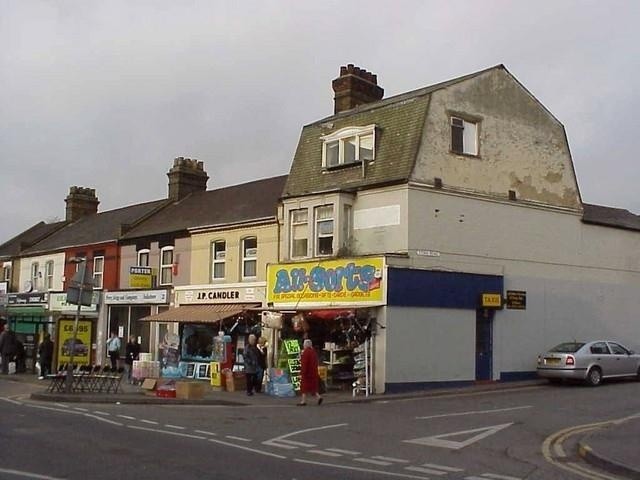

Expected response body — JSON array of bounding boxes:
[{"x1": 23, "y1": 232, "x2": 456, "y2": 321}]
[{"x1": 61, "y1": 254, "x2": 94, "y2": 394}]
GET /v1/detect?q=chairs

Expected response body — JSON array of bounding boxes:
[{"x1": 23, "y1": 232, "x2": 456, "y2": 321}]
[{"x1": 42, "y1": 360, "x2": 127, "y2": 396}]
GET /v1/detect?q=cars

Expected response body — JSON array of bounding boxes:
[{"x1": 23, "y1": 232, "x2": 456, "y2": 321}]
[
  {"x1": 536, "y1": 339, "x2": 640, "y2": 384},
  {"x1": 61, "y1": 337, "x2": 87, "y2": 357}
]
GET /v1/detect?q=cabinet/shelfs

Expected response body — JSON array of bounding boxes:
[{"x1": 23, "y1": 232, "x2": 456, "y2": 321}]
[{"x1": 319, "y1": 337, "x2": 373, "y2": 398}]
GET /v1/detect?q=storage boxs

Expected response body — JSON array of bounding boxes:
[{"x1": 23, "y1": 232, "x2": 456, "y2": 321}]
[{"x1": 140, "y1": 363, "x2": 297, "y2": 402}]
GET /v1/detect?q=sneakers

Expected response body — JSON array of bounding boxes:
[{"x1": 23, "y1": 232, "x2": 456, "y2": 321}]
[
  {"x1": 318, "y1": 397, "x2": 323, "y2": 406},
  {"x1": 296, "y1": 403, "x2": 307, "y2": 406}
]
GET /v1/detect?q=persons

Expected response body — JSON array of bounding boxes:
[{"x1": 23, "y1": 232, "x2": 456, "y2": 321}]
[
  {"x1": 296, "y1": 339, "x2": 323, "y2": 406},
  {"x1": 39, "y1": 333, "x2": 54, "y2": 379},
  {"x1": 256, "y1": 337, "x2": 269, "y2": 393},
  {"x1": 13, "y1": 340, "x2": 26, "y2": 373},
  {"x1": 105, "y1": 330, "x2": 121, "y2": 372},
  {"x1": 0, "y1": 323, "x2": 16, "y2": 374},
  {"x1": 243, "y1": 334, "x2": 261, "y2": 396},
  {"x1": 125, "y1": 334, "x2": 141, "y2": 384}
]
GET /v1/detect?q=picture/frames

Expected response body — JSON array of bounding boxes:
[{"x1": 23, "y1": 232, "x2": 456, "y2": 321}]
[{"x1": 284, "y1": 339, "x2": 304, "y2": 392}]
